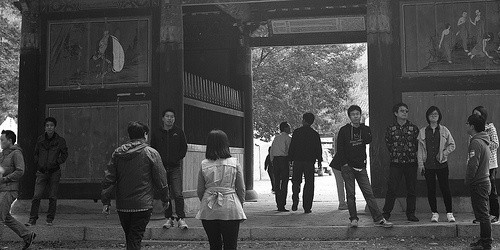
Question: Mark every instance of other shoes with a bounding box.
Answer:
[491,217,499,223]
[382,212,391,220]
[338,204,348,210]
[278,205,290,211]
[292,205,297,211]
[304,209,311,213]
[472,219,480,224]
[408,215,419,222]
[470,242,491,250]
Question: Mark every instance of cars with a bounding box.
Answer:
[315,149,333,175]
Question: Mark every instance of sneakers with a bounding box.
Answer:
[446,213,456,222]
[430,212,440,222]
[373,218,393,228]
[350,219,358,227]
[25,218,36,226]
[178,219,188,230]
[163,217,175,229]
[46,219,53,226]
[22,231,37,250]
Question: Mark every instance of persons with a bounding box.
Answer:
[0,130,37,250]
[288,113,322,213]
[151,109,188,229]
[100,120,171,250]
[196,130,247,250]
[383,103,420,222]
[328,106,393,227]
[265,147,276,193]
[25,117,67,227]
[416,106,455,223]
[465,106,500,250]
[270,121,292,212]
